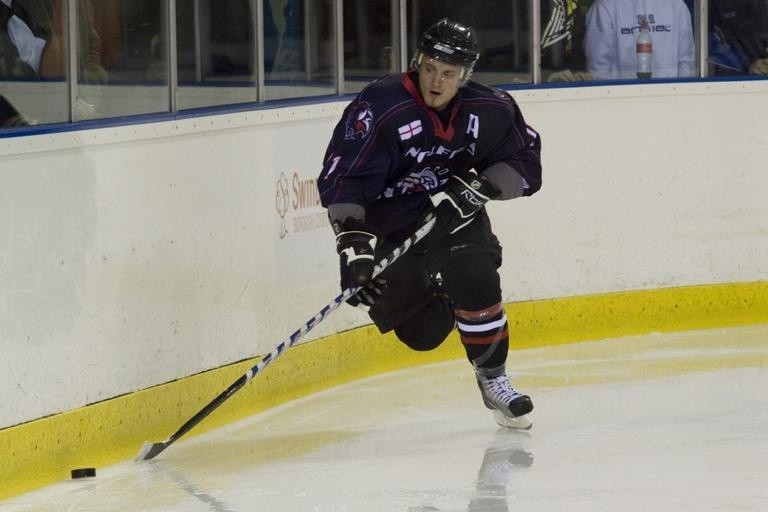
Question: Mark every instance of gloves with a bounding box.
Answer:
[337,230,387,307]
[416,169,500,244]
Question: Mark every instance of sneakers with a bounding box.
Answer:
[474,372,532,418]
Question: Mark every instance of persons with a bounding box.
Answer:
[319,18,552,417]
[540,0,768,88]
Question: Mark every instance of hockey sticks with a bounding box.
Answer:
[135,217,436,462]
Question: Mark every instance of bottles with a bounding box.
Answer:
[636,22,653,79]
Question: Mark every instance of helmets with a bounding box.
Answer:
[410,18,481,89]
